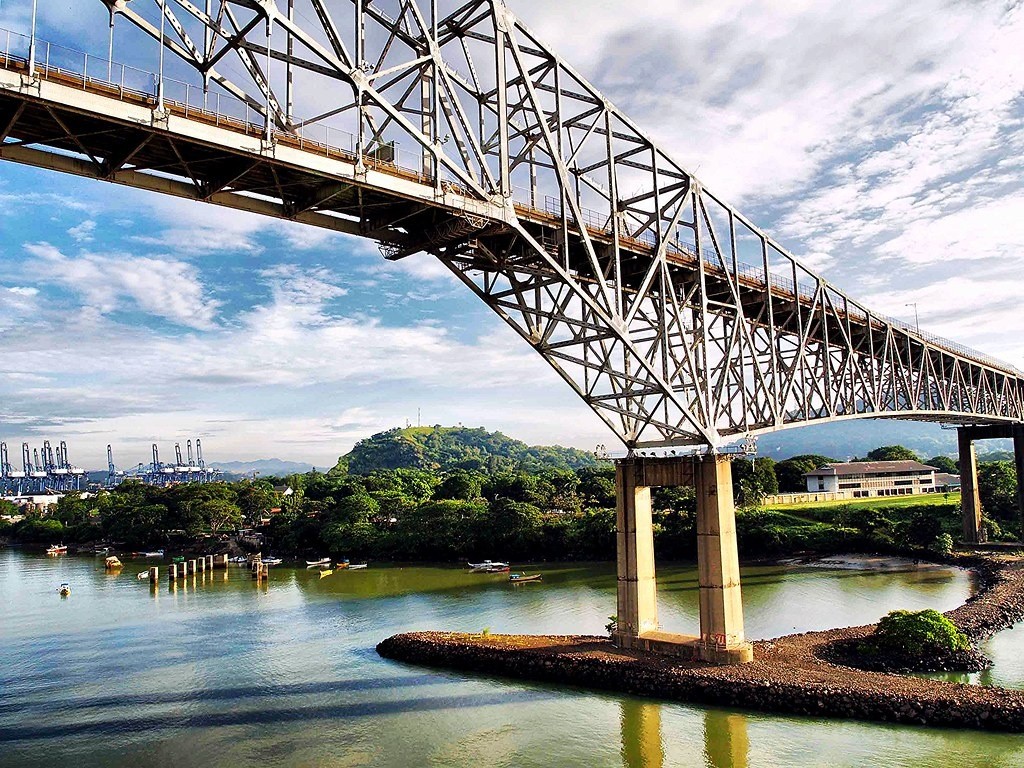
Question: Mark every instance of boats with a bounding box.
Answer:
[468,560,511,572]
[337,563,368,570]
[104,555,124,571]
[229,556,283,567]
[306,556,332,565]
[509,571,543,582]
[59,582,70,597]
[46,544,68,552]
